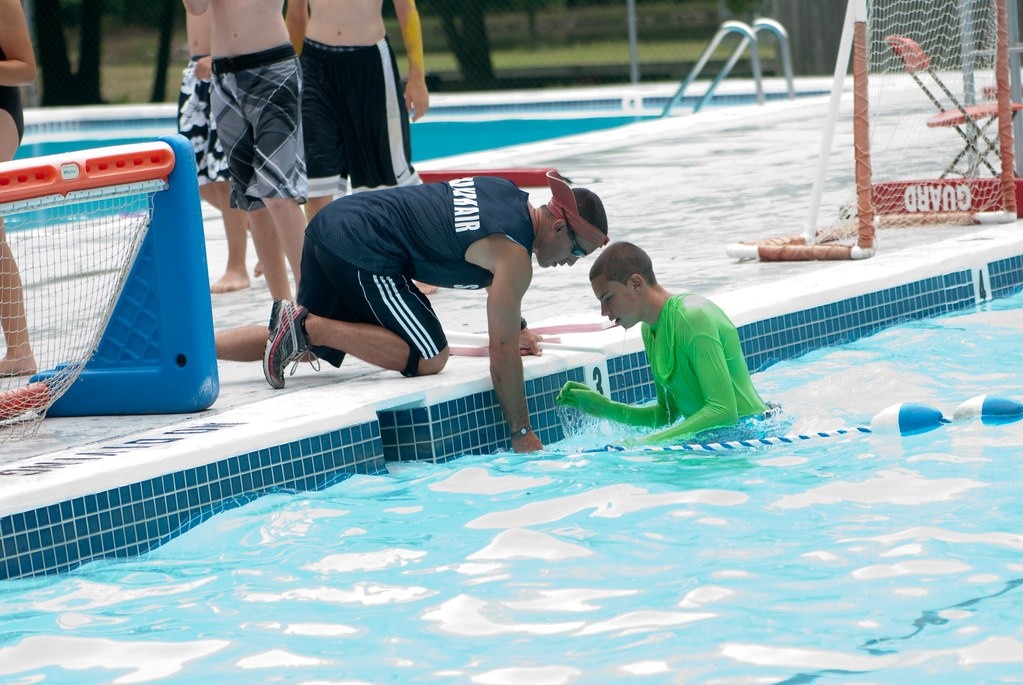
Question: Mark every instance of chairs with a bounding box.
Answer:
[886,33,1022,180]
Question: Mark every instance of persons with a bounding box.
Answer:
[177,0,264,293]
[298,0,429,223]
[217,169,611,453]
[183,0,310,302]
[556,242,770,446]
[0,0,37,377]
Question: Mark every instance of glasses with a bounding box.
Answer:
[561,207,587,257]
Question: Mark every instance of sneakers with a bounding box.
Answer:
[261,298,320,389]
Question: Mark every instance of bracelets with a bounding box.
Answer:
[510,425,532,438]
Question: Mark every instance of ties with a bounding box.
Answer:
[546,170,610,248]
